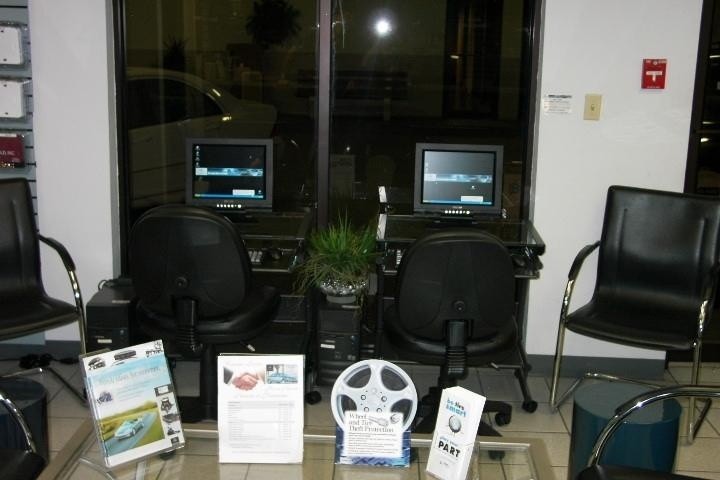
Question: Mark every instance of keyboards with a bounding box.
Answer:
[395,248,404,269]
[248,250,265,263]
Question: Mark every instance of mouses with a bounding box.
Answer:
[269,248,283,260]
[510,253,526,267]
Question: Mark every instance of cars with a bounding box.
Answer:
[266,372,296,384]
[126,66,278,208]
[115,418,144,439]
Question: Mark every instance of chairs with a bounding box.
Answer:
[549,182,720,480]
[377,233,517,459]
[131,207,284,423]
[0,178,92,455]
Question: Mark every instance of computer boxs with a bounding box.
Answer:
[83,283,133,400]
[313,303,361,387]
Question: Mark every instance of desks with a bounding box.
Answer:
[372,209,545,415]
[40,413,557,480]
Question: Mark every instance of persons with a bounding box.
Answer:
[234,369,266,389]
[224,367,258,391]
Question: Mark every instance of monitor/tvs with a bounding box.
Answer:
[413,142,504,229]
[183,137,274,223]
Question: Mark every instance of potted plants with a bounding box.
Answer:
[307,218,371,303]
